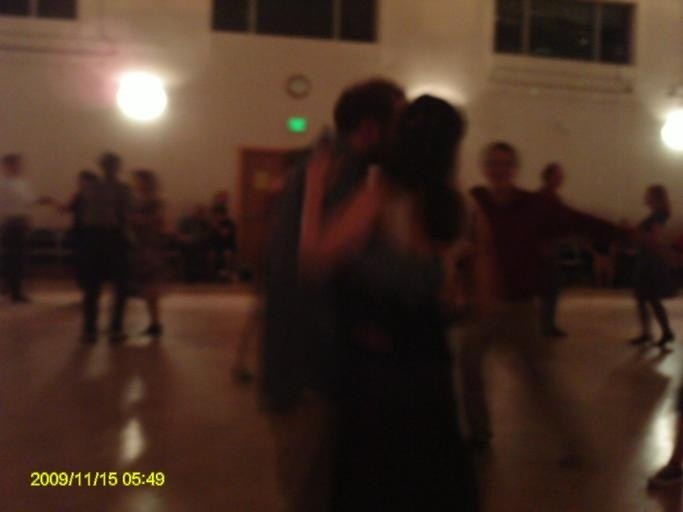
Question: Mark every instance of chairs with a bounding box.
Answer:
[26,225,181,276]
